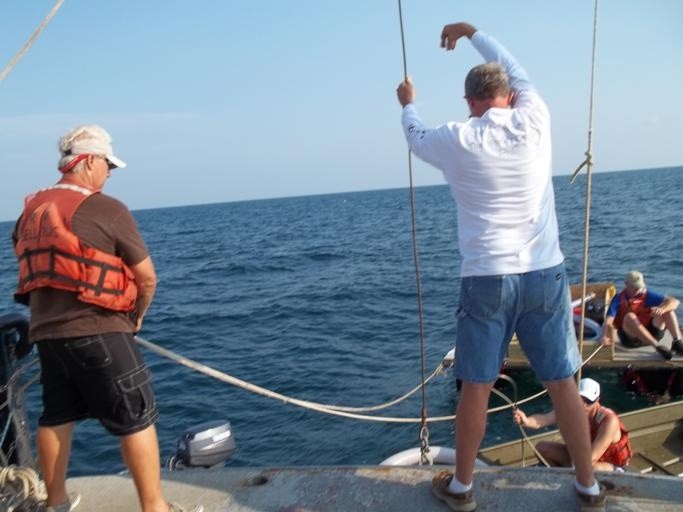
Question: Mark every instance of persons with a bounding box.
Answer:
[512,378,633,473]
[395,22,606,511]
[599,270,682,361]
[11,123,206,511]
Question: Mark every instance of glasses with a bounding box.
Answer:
[582,397,597,405]
[98,156,118,170]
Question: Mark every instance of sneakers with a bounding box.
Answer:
[656,345,673,361]
[432,469,476,512]
[46,492,82,512]
[671,340,683,355]
[575,483,609,512]
[168,502,204,512]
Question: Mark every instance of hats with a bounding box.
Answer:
[576,377,601,401]
[624,270,646,290]
[60,125,127,170]
